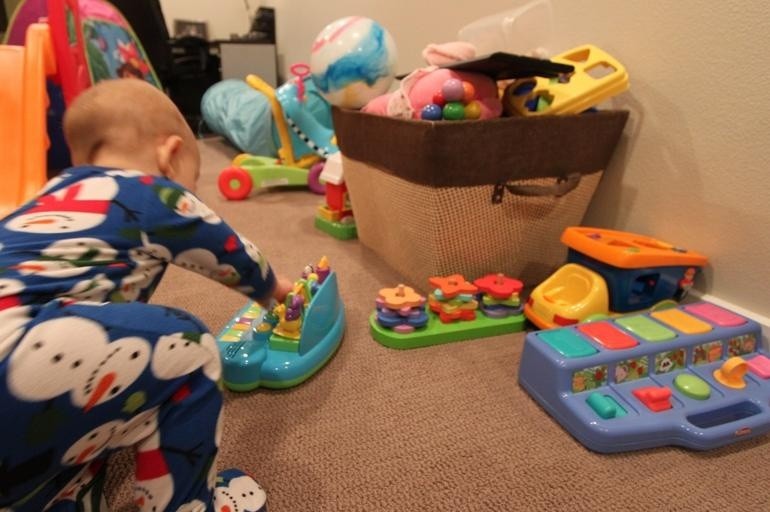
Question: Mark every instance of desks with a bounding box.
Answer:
[168,34,281,122]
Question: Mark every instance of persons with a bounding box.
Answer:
[0,76,293,512]
